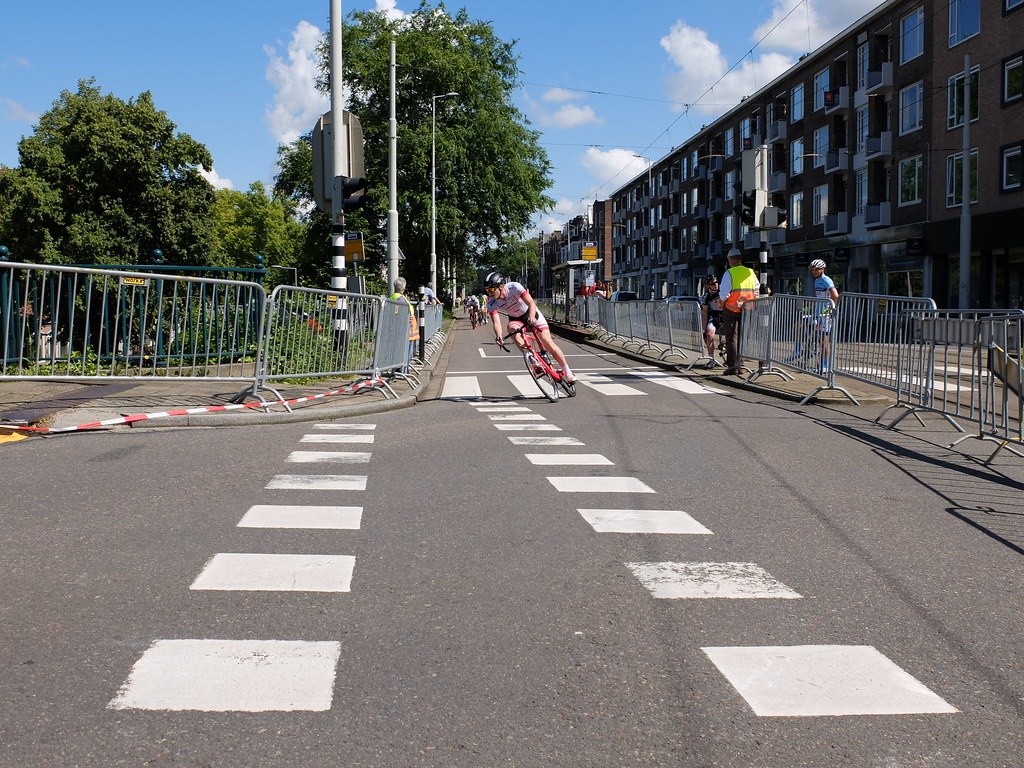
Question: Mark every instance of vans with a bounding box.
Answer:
[609,290,641,301]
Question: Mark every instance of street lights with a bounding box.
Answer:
[428,91,460,303]
[631,154,651,301]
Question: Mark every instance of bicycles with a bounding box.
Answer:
[462,304,489,329]
[498,317,576,401]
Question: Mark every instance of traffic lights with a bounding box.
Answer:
[765,206,787,229]
[742,188,756,225]
[341,176,368,216]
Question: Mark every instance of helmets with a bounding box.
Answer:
[808,259,827,269]
[483,272,504,289]
[703,274,717,283]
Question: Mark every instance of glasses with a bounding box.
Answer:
[485,287,495,292]
[709,281,720,286]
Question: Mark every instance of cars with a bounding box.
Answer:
[664,294,704,312]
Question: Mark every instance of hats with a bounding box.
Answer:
[727,248,741,258]
[471,295,476,300]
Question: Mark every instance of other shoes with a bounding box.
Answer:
[702,361,717,370]
[723,370,738,376]
[812,367,821,375]
[563,371,575,384]
[820,367,830,374]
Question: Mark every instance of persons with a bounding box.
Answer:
[699,273,725,369]
[424,282,444,306]
[719,248,761,376]
[782,258,839,374]
[388,277,420,376]
[462,293,490,329]
[486,271,577,383]
